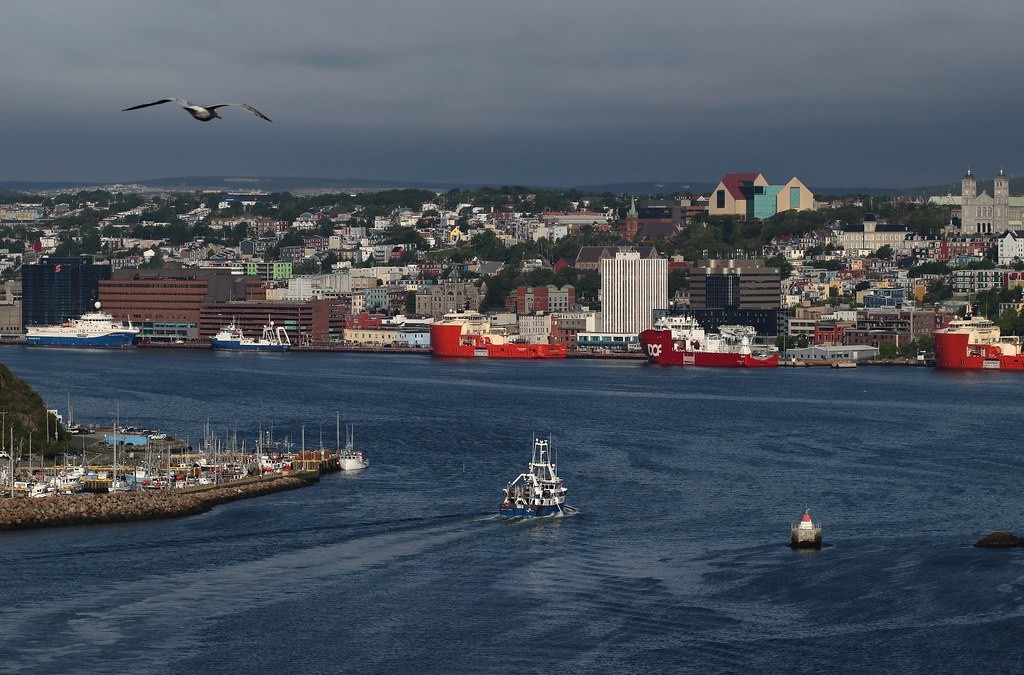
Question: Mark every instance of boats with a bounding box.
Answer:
[791,504,823,550]
[637,303,779,368]
[0,389,290,499]
[208,314,292,352]
[930,303,1024,372]
[337,423,369,471]
[429,300,568,359]
[499,430,568,520]
[24,302,141,350]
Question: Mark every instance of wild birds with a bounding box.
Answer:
[124,98,274,126]
